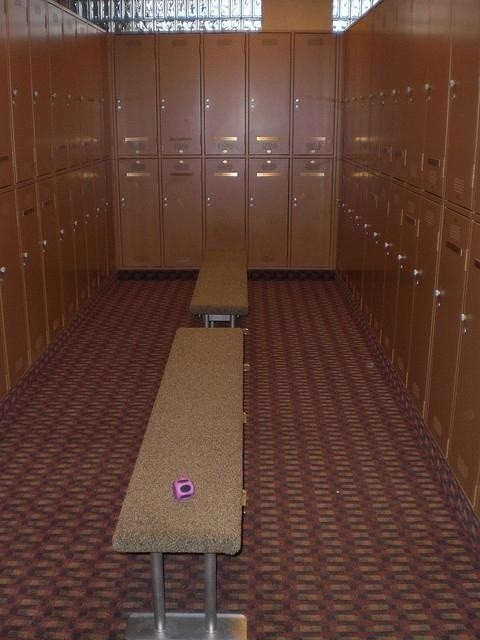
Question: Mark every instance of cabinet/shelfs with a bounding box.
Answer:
[293,33,339,157]
[110,34,159,158]
[155,34,203,155]
[248,159,292,269]
[246,34,292,157]
[204,158,248,268]
[116,156,159,269]
[1,0,109,399]
[341,0,479,520]
[202,34,247,157]
[289,158,336,269]
[161,156,203,268]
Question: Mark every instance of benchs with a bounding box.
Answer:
[111,328,249,640]
[189,271,249,328]
